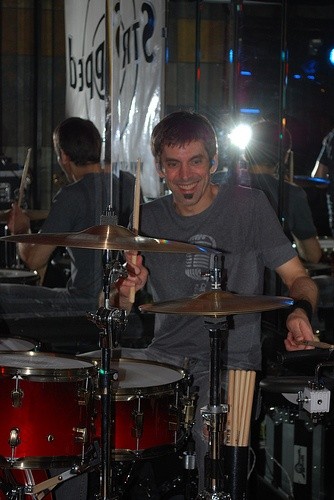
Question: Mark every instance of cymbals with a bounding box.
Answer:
[141,294,293,316]
[0,209,50,223]
[293,176,329,188]
[0,223,222,255]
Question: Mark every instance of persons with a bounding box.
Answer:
[210,120,321,263]
[99,111,319,500]
[12,117,144,353]
[312,129,334,240]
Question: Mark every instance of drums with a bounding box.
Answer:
[304,263,332,276]
[293,238,334,266]
[77,356,194,462]
[0,352,94,469]
[0,337,35,352]
[311,275,334,339]
[256,374,334,500]
[0,267,40,286]
